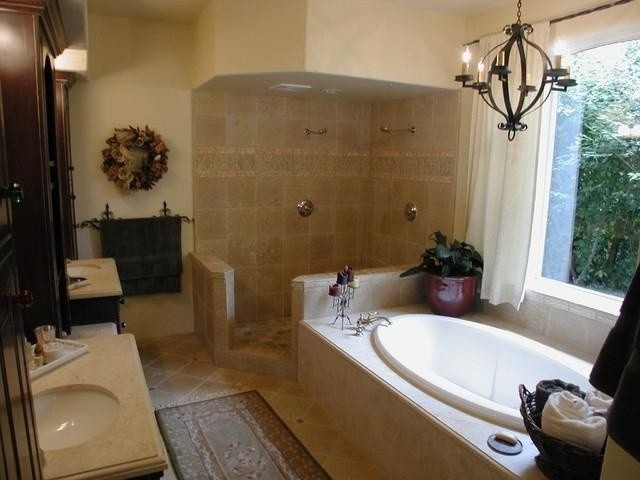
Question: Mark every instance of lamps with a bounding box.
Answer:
[451,0,579,143]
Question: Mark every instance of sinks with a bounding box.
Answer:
[32,382,119,450]
[67,262,101,288]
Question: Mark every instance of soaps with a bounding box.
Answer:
[493,429,517,445]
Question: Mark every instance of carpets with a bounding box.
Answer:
[154,387,333,480]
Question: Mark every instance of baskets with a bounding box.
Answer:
[518,383,605,480]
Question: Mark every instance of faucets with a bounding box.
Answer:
[354,315,392,333]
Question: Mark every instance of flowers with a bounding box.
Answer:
[102,124,169,192]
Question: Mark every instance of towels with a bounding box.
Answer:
[97,216,186,298]
[534,375,610,451]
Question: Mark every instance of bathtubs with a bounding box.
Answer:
[373,312,597,430]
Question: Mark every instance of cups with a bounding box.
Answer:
[32,324,57,352]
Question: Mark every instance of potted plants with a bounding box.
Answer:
[405,228,484,317]
